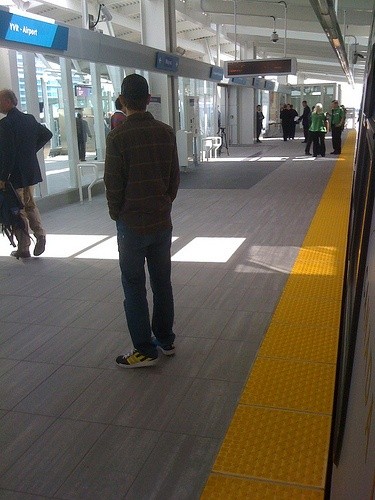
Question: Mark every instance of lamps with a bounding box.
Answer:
[90,3,111,29]
[351,52,359,64]
[13,0,30,10]
[176,47,185,55]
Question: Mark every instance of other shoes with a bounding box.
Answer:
[257,139,260,142]
[302,140,307,143]
[94,156,97,160]
[322,154,325,157]
[330,151,341,154]
[81,159,87,161]
[313,154,317,157]
[305,152,312,155]
[284,138,287,141]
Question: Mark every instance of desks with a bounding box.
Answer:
[177,131,194,168]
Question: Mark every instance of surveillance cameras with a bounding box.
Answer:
[271,33,279,43]
[100,3,112,21]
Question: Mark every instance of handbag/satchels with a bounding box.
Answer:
[0,187,26,229]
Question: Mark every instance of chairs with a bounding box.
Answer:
[200,137,222,161]
[78,160,106,199]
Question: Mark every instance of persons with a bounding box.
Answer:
[103,74,179,368]
[305,103,328,157]
[256,105,264,143]
[104,112,112,137]
[76,113,92,161]
[298,101,312,142]
[280,104,298,140]
[325,100,346,154]
[111,95,127,131]
[0,89,54,257]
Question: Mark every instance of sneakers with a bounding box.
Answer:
[152,336,175,355]
[11,251,31,258]
[34,236,46,256]
[116,348,159,367]
